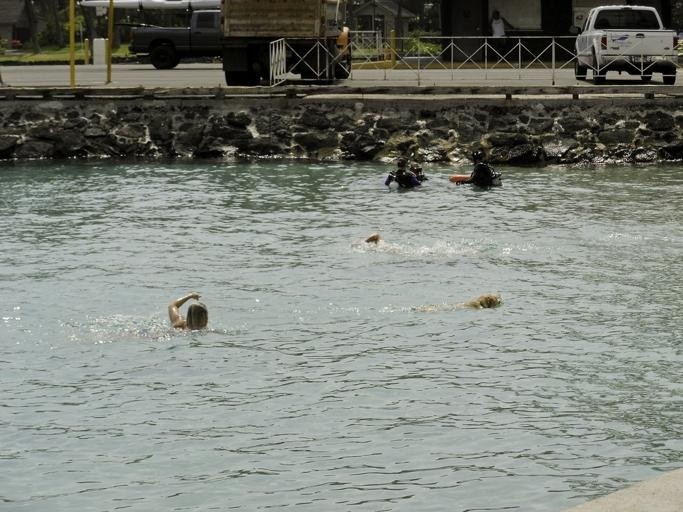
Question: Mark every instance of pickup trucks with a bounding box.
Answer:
[572,7,679,85]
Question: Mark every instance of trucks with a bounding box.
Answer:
[125,6,223,73]
[217,0,357,87]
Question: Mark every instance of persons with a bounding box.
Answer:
[350,234,379,246]
[168,292,208,329]
[410,163,427,181]
[385,158,421,189]
[463,150,492,186]
[490,10,513,63]
